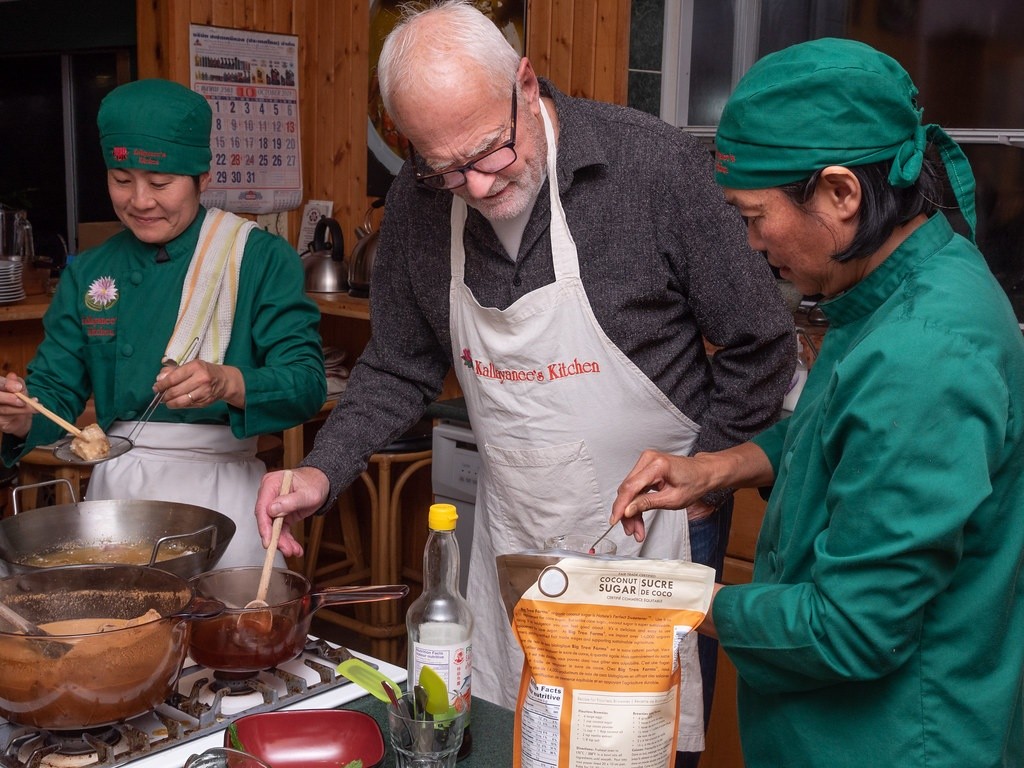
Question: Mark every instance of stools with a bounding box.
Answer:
[13,393,102,508]
[298,423,434,667]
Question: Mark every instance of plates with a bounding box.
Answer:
[0,260,26,302]
[367,0,520,176]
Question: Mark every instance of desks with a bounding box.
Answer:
[0,255,371,389]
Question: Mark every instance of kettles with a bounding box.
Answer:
[348,201,385,298]
[302,219,348,294]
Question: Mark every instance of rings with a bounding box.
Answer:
[188,394,193,401]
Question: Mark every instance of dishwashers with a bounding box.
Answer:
[430,421,480,597]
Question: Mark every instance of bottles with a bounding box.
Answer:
[406,503,472,761]
[45,269,60,295]
[0,209,34,263]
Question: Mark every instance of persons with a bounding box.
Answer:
[255,0,801,768]
[610,36,1024,767]
[0,76,329,607]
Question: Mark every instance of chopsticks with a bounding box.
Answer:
[14,392,88,443]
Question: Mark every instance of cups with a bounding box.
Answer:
[544,534,619,555]
[387,689,468,767]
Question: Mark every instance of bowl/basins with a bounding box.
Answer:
[224,709,384,768]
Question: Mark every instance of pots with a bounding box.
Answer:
[0,479,235,581]
[186,566,408,674]
[0,562,224,730]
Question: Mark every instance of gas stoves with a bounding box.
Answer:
[0,632,408,768]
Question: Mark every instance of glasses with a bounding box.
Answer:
[408,83,518,192]
